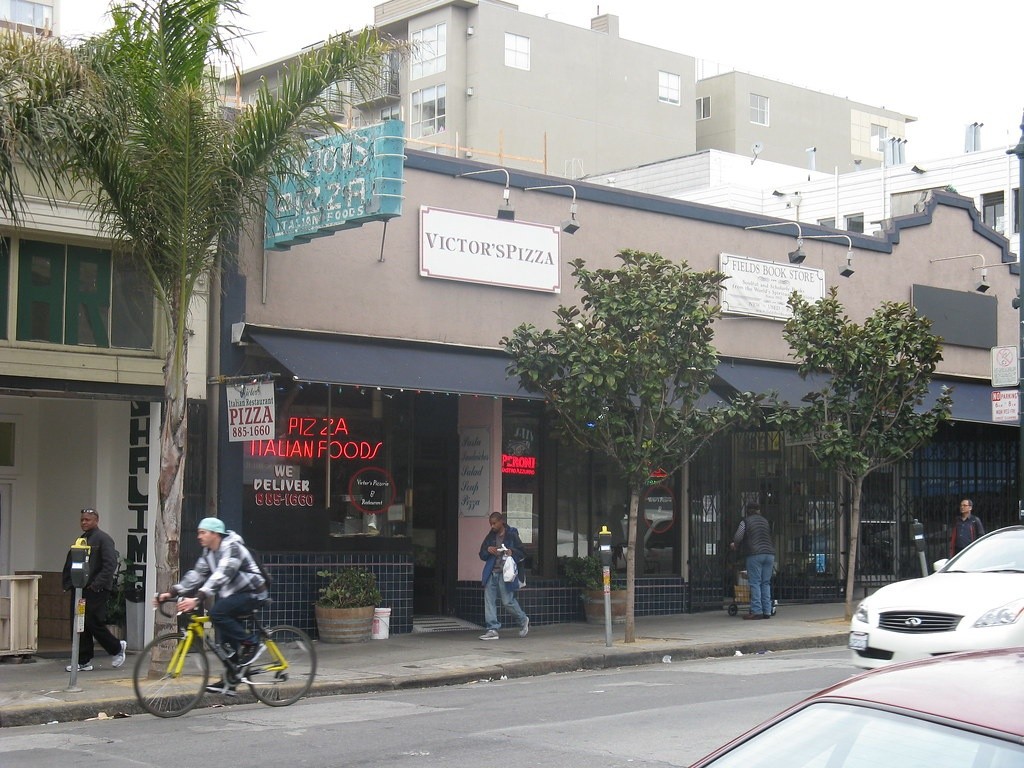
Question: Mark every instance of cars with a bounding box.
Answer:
[507,510,588,552]
[616,509,701,569]
[687,647,1024,768]
[850,525,1024,670]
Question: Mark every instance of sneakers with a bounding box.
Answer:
[519,616,530,638]
[206,680,238,696]
[238,642,268,668]
[478,629,499,639]
[112,639,128,668]
[66,660,93,671]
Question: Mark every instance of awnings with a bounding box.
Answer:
[246,330,737,433]
[712,361,1021,427]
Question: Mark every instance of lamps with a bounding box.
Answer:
[454,168,515,220]
[971,262,1020,298]
[524,184,580,235]
[797,234,856,278]
[745,221,806,264]
[930,254,991,294]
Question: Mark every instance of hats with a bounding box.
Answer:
[198,517,229,535]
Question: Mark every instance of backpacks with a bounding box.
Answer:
[232,541,271,585]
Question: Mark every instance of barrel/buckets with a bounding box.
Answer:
[371,607,392,639]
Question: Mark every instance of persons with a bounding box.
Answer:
[62,510,127,671]
[153,517,270,695]
[952,499,985,555]
[597,504,625,568]
[478,512,529,640]
[730,501,776,620]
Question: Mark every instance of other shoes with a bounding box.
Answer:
[742,611,771,620]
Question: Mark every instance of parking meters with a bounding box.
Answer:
[598,526,613,646]
[65,537,92,692]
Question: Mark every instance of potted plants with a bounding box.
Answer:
[311,568,382,644]
[562,555,627,625]
[93,549,138,643]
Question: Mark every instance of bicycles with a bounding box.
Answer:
[133,593,317,719]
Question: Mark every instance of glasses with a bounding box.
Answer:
[960,504,972,507]
[81,509,98,517]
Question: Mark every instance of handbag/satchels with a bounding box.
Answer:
[496,542,518,582]
